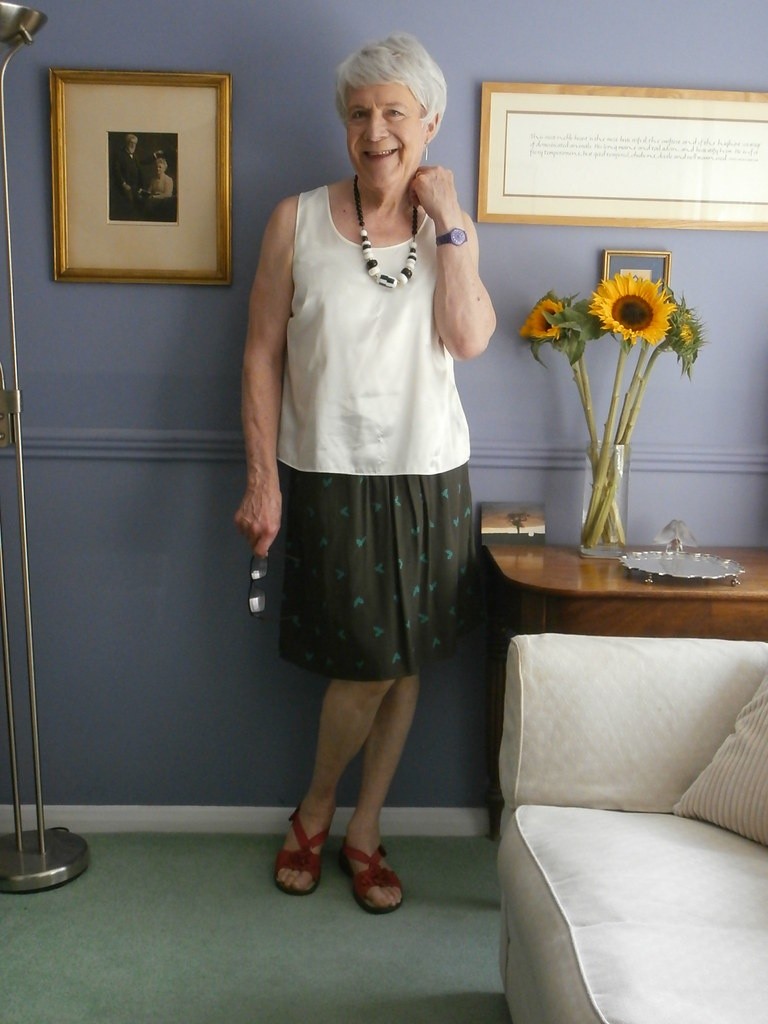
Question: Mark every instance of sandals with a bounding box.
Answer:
[274,805,406,916]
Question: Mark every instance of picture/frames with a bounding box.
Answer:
[602,250,672,304]
[478,84,768,231]
[46,69,231,288]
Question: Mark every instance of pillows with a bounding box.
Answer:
[669,663,768,850]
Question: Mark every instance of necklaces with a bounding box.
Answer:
[353,174,417,289]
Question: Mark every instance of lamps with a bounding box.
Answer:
[0,2,90,894]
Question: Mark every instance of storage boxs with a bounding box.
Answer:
[480,503,545,545]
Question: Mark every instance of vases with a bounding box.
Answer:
[578,445,626,557]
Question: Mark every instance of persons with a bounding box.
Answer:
[232,33,499,915]
[112,133,173,222]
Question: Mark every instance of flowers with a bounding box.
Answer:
[521,279,701,441]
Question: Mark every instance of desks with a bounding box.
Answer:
[489,543,768,637]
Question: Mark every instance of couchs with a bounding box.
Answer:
[496,634,768,1024]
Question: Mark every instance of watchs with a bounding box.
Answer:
[436,228,468,247]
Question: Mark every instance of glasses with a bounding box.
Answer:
[247,550,271,624]
